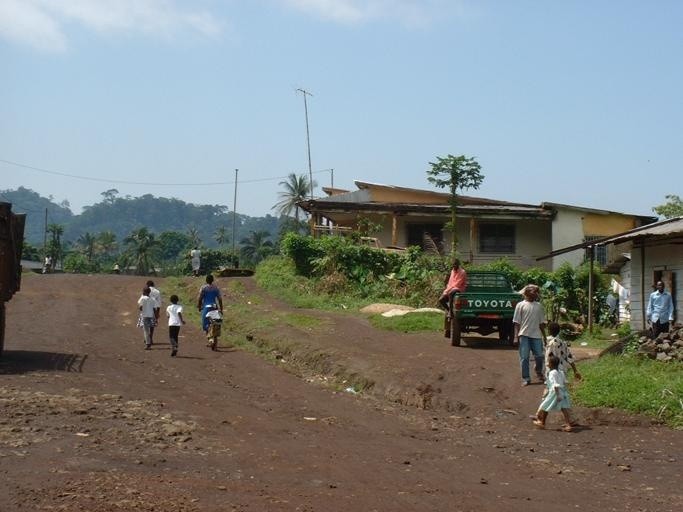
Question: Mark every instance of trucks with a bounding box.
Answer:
[442,269,521,348]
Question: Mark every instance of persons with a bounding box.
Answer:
[438,258,467,319]
[529,322,582,427]
[137,280,160,350]
[196,274,223,336]
[512,285,547,387]
[532,355,572,432]
[191,246,201,276]
[646,280,675,339]
[166,294,186,356]
[45,254,52,273]
[114,263,119,271]
